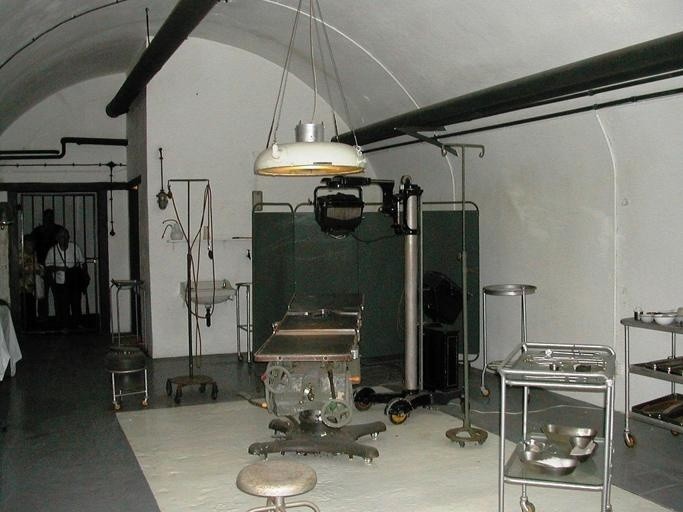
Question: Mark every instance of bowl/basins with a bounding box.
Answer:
[654,314,674,325]
[641,313,654,323]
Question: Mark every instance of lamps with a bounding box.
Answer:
[254,1,368,176]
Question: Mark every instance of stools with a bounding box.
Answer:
[104,355,151,410]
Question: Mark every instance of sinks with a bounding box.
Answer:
[180,278,238,304]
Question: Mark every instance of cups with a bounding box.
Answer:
[634,312,644,321]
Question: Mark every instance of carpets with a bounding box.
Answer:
[116,385,673,511]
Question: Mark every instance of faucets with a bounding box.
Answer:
[223,279,226,288]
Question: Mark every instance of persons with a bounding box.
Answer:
[19,233,44,330]
[33,208,65,317]
[45,228,86,316]
